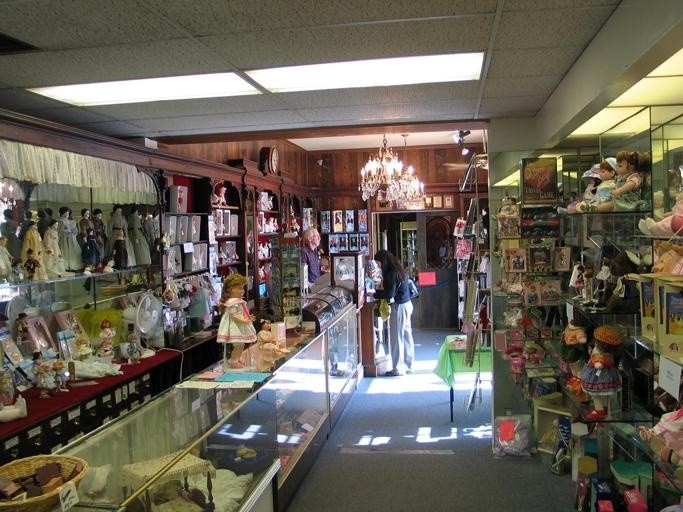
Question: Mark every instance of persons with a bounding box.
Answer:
[328,325,345,376]
[161,183,278,368]
[493,149,683,512]
[367,250,414,376]
[0,182,283,422]
[301,228,331,294]
[1,206,161,406]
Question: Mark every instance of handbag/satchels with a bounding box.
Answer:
[409,278,419,301]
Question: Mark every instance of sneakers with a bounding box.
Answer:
[385,370,396,376]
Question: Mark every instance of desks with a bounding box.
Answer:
[445,332,490,423]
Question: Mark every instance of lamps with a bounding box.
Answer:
[357,134,425,211]
[454,129,472,156]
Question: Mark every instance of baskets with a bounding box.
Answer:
[0,454,89,511]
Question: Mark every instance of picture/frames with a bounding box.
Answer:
[375,192,455,212]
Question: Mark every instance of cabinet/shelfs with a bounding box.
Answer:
[0,106,369,512]
[489,106,683,512]
